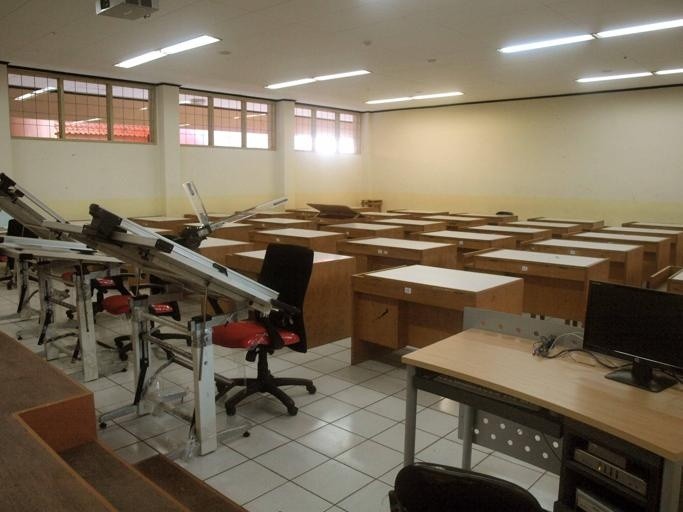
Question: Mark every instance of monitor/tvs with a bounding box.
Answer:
[582,279,683,393]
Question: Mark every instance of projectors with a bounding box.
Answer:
[95,0,160,22]
